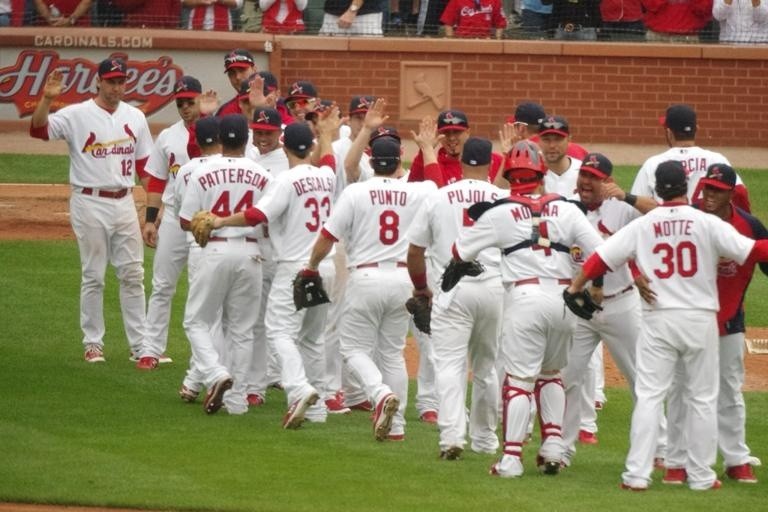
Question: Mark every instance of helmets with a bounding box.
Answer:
[502,141,547,195]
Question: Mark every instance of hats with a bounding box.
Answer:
[98,57,131,80]
[579,153,613,178]
[461,138,492,166]
[658,104,736,194]
[195,71,342,152]
[504,103,546,125]
[437,110,468,133]
[224,48,255,73]
[169,76,202,101]
[537,116,569,137]
[348,95,401,176]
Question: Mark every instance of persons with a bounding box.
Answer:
[629,165,768,484]
[623,103,752,217]
[444,135,604,479]
[0,1,768,48]
[566,160,768,490]
[211,47,258,118]
[140,73,645,455]
[29,57,176,367]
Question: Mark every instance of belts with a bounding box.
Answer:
[603,285,633,299]
[515,278,571,286]
[357,262,407,268]
[208,236,257,242]
[81,187,133,199]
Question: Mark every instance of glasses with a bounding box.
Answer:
[177,101,194,107]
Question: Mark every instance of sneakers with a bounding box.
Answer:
[725,464,759,484]
[620,458,722,491]
[439,434,567,475]
[578,430,599,445]
[84,344,106,362]
[420,411,438,423]
[282,390,406,441]
[595,402,603,410]
[179,376,265,415]
[136,357,158,369]
[128,350,173,363]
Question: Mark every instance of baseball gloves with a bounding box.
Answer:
[295,272,330,310]
[563,289,604,318]
[441,257,484,290]
[191,209,215,245]
[406,295,434,335]
